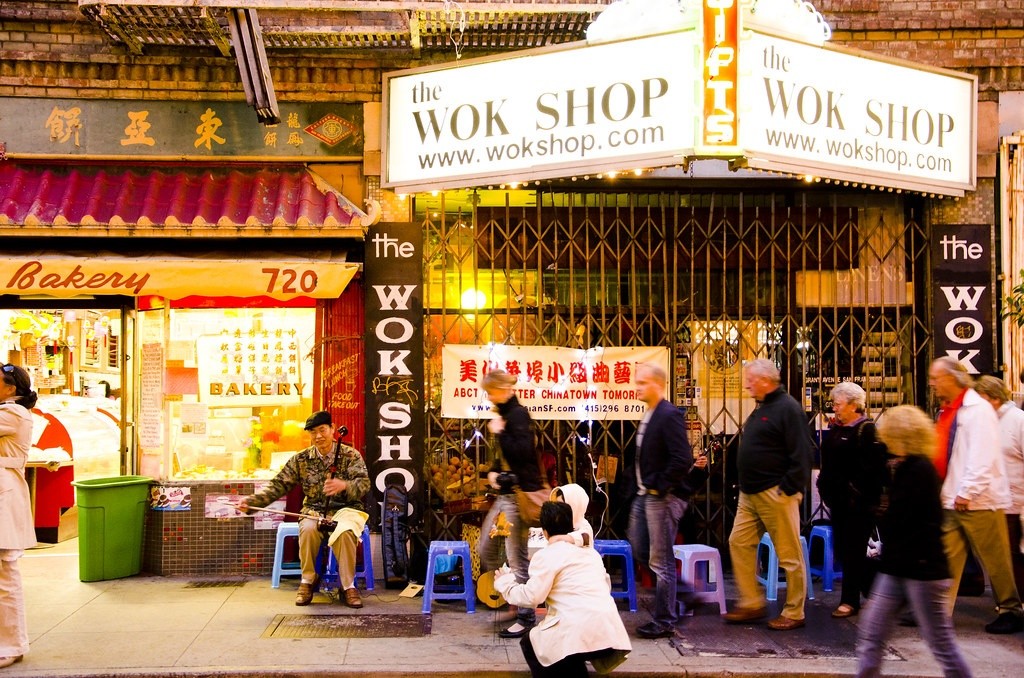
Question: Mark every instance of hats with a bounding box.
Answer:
[303,411,331,431]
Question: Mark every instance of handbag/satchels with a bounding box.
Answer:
[381,484,409,589]
[851,485,893,518]
[517,489,552,527]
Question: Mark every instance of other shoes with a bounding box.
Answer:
[831,603,859,617]
[985,611,1024,633]
[899,612,920,627]
[0,655,23,667]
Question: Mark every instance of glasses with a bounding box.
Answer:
[3,366,17,381]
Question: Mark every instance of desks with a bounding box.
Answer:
[24,461,75,549]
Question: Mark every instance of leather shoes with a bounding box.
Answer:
[767,615,804,629]
[498,623,535,637]
[635,622,674,638]
[722,608,765,621]
[339,586,362,607]
[296,573,319,605]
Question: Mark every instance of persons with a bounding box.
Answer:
[629,364,708,638]
[483,369,548,638]
[548,484,594,547]
[494,501,632,678]
[238,411,370,608]
[816,382,887,617]
[856,404,971,678]
[99,380,114,400]
[0,363,37,667]
[722,360,811,628]
[930,357,1024,634]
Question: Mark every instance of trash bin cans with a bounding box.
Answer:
[70,475,153,583]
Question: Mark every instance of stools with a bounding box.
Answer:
[672,544,727,615]
[422,541,476,614]
[755,532,816,600]
[809,526,843,592]
[593,540,637,611]
[271,522,374,590]
